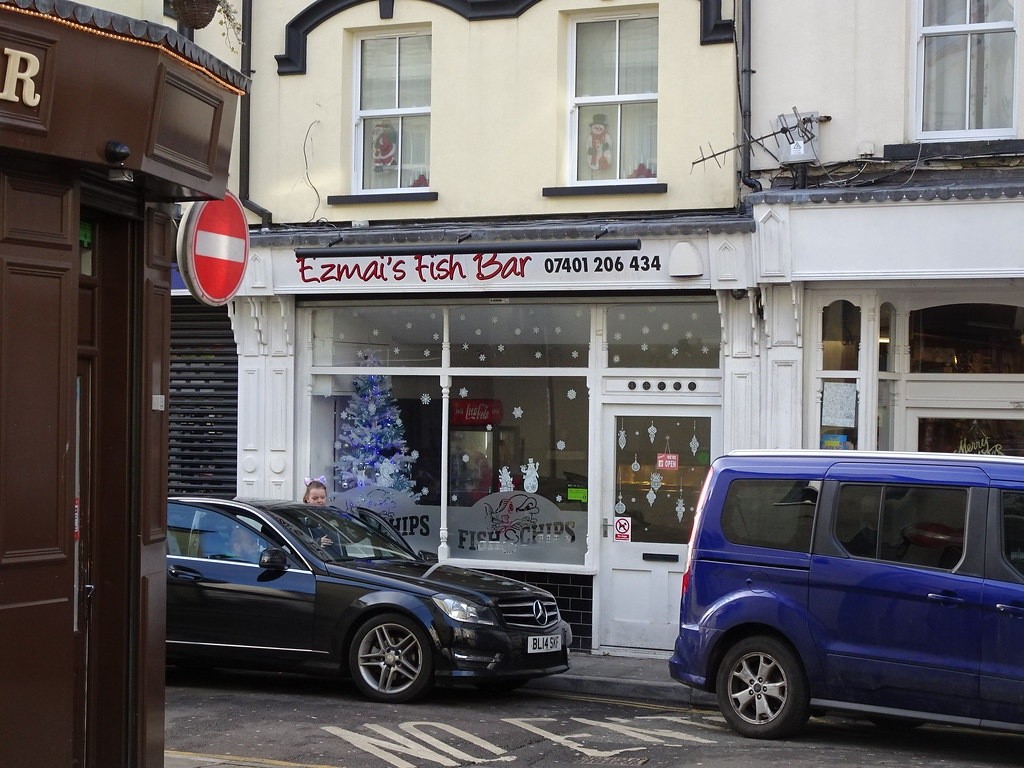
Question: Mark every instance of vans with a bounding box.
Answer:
[667,450,1024,742]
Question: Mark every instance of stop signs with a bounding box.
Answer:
[176,187,250,307]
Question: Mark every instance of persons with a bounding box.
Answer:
[472,441,522,503]
[303,480,370,559]
[225,528,262,565]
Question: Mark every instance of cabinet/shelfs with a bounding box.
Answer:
[450,426,520,506]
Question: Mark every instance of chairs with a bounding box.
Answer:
[168,526,234,559]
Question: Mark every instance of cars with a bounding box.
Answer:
[164,491,574,703]
